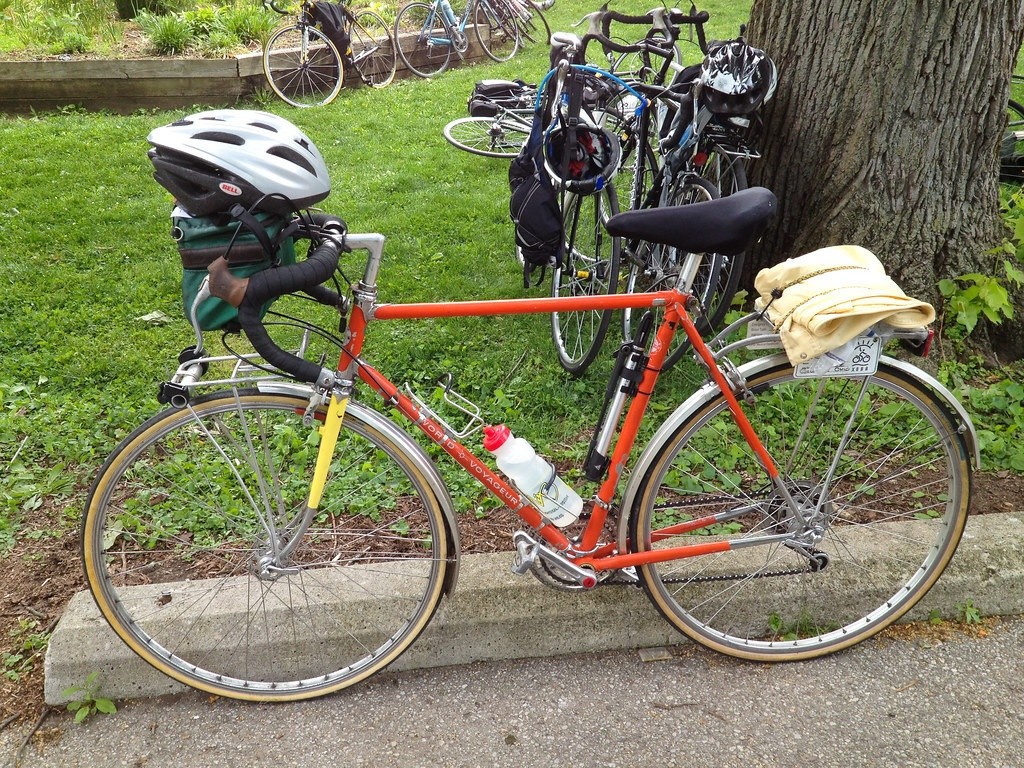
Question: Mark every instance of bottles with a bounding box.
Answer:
[483,424,582,528]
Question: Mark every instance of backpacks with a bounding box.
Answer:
[168,200,297,332]
[507,63,584,289]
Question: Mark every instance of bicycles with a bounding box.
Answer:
[395,1,764,379]
[263,0,397,108]
[1001,74,1024,186]
[80,185,982,704]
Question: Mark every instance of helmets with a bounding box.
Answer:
[541,124,623,196]
[146,108,332,226]
[698,40,779,116]
